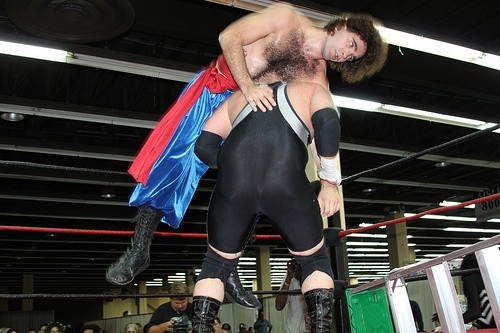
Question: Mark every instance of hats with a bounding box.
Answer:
[222,323,231,330]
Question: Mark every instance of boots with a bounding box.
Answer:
[303,288,335,333]
[225,264,261,309]
[191,295,222,333]
[106,204,164,286]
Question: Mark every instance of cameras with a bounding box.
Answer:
[172,315,191,333]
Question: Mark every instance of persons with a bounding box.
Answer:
[144,281,215,333]
[0,316,272,333]
[389,253,497,333]
[192,80,341,333]
[107,5,387,311]
